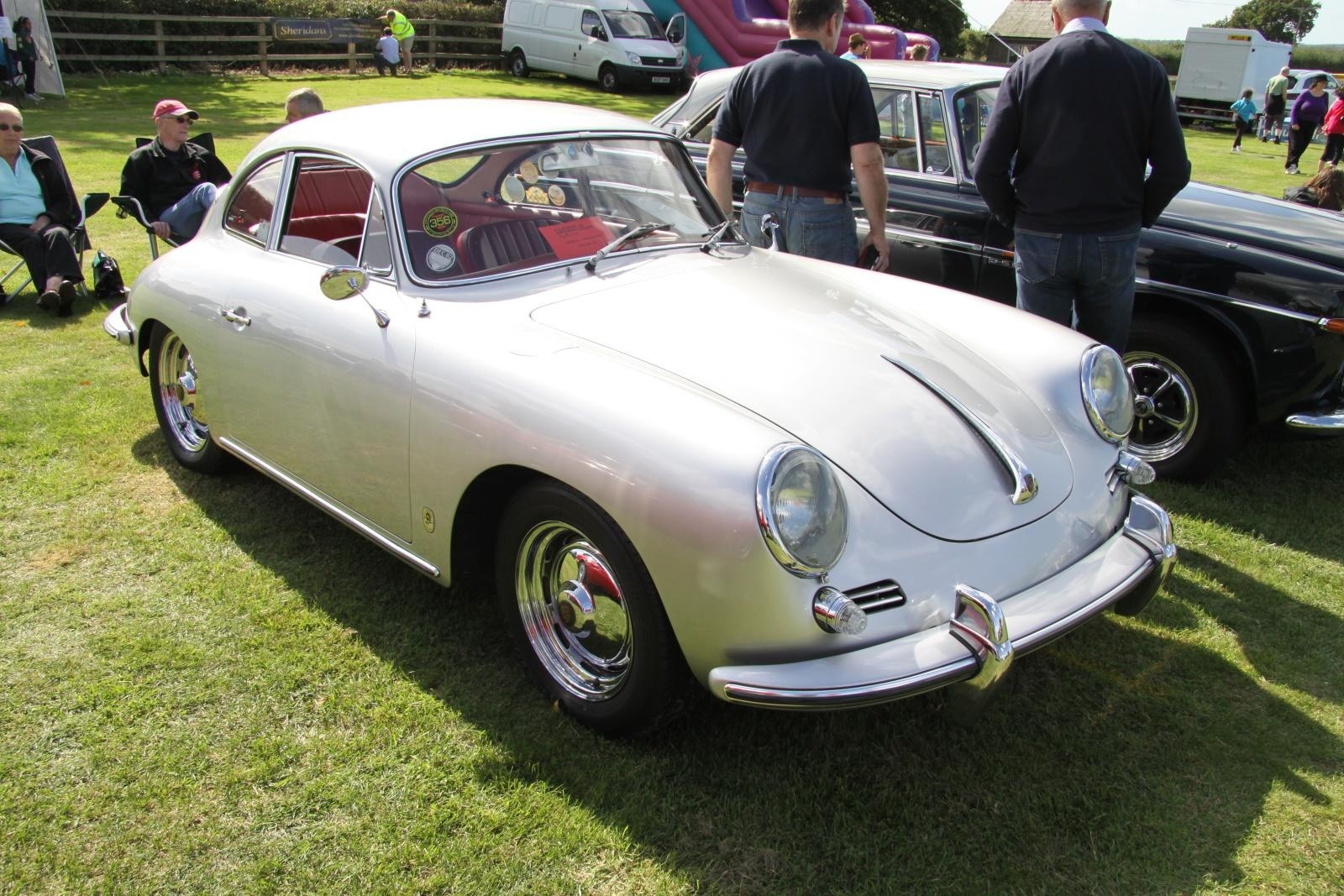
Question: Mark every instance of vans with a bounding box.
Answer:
[502,0,688,93]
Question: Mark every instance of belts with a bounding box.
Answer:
[747,180,846,199]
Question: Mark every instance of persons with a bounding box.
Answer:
[1230,89,1257,151]
[900,45,933,141]
[1263,67,1290,144]
[1283,166,1344,212]
[374,9,415,76]
[973,0,1191,354]
[1318,87,1344,171]
[13,17,45,101]
[1285,75,1330,175]
[120,100,232,240]
[840,33,871,59]
[708,0,890,273]
[286,89,330,123]
[1,102,85,317]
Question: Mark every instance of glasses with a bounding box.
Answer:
[0,123,24,133]
[160,116,194,126]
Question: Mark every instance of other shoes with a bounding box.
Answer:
[1274,138,1280,144]
[36,290,61,313]
[1237,145,1242,152]
[1262,137,1267,142]
[1231,149,1240,154]
[56,280,76,317]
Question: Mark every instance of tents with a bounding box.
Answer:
[0,0,65,97]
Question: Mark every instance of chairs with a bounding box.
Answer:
[456,217,563,279]
[111,130,228,260]
[314,229,449,282]
[0,136,110,303]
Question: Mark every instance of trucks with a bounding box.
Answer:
[1173,26,1293,132]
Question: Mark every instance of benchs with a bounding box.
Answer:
[241,167,442,259]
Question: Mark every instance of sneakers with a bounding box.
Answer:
[1285,164,1300,174]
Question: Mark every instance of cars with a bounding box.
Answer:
[104,97,1178,736]
[591,59,1343,477]
[1282,69,1341,117]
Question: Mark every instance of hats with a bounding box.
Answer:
[153,99,200,121]
[848,33,866,44]
[1313,74,1330,83]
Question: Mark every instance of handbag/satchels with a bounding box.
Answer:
[92,248,126,299]
